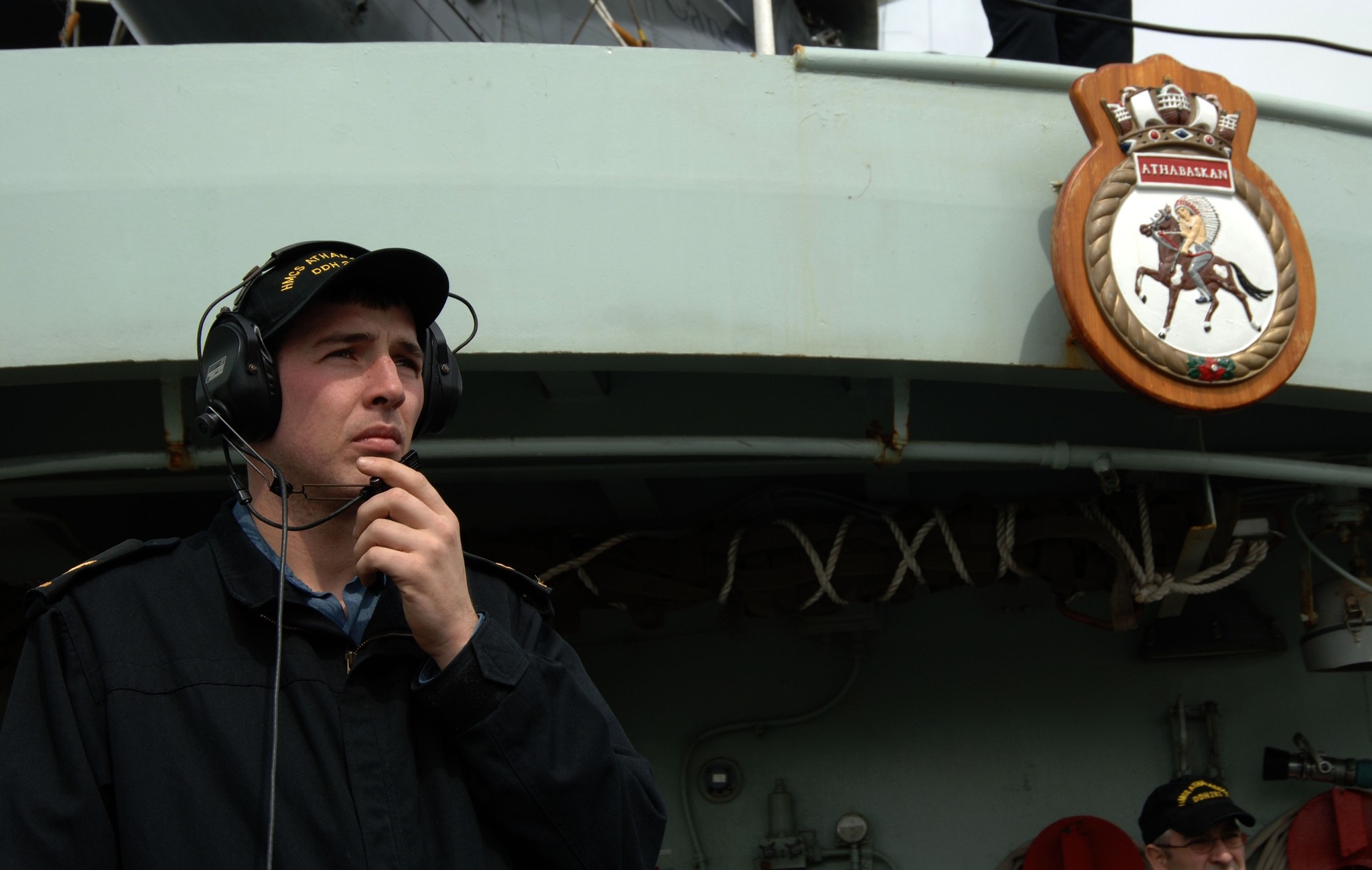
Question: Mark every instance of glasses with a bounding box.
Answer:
[1159,827,1243,855]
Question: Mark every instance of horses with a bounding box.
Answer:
[1135,203,1274,339]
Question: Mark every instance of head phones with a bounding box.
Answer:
[193,240,464,446]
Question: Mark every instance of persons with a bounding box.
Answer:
[0,240,668,870]
[1137,774,1246,870]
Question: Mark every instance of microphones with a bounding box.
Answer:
[220,441,422,532]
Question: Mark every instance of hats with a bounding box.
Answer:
[241,245,450,348]
[1138,775,1255,846]
